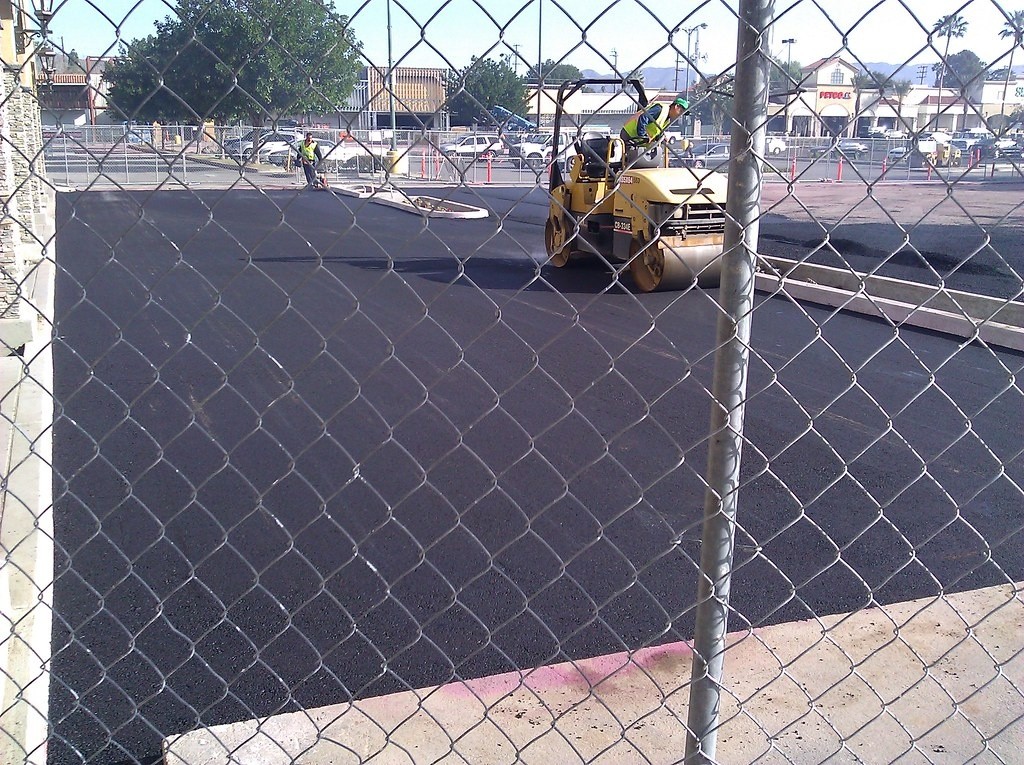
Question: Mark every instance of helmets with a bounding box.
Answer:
[672,98,690,116]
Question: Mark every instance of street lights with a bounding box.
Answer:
[782,37,797,134]
[674,22,708,138]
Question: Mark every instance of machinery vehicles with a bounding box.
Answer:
[545,76,729,295]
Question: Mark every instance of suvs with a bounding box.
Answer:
[432,135,504,163]
[664,125,683,144]
[236,131,308,164]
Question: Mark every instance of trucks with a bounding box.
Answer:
[507,131,615,171]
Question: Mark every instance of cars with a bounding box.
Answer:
[860,125,1024,170]
[810,142,869,160]
[766,136,786,155]
[222,130,252,154]
[668,143,732,168]
[262,138,347,163]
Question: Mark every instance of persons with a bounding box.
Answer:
[620,98,690,145]
[297,132,325,187]
[68,48,80,66]
